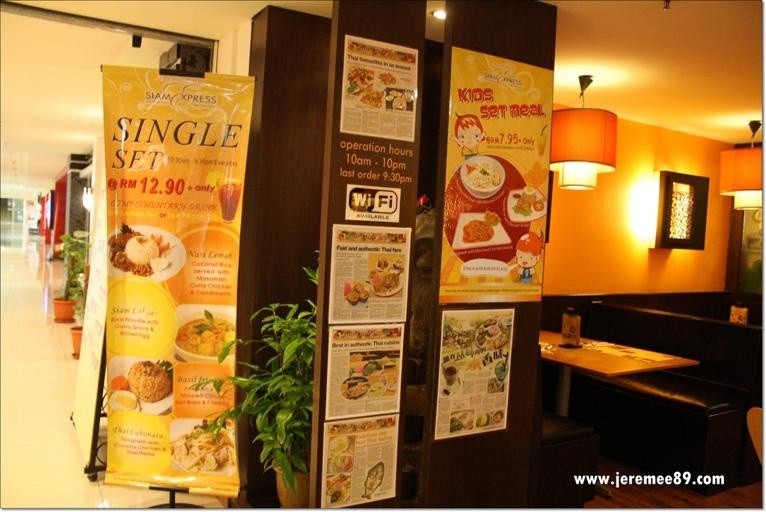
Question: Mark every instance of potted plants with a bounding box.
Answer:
[66,278,87,360]
[48,230,92,324]
[182,249,322,507]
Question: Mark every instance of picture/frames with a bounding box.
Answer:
[547,169,554,244]
[652,171,709,250]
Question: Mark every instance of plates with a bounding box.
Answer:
[168,435,234,473]
[505,186,547,224]
[369,270,404,297]
[340,367,397,400]
[106,224,187,283]
[451,210,512,251]
[324,435,352,506]
[106,364,173,416]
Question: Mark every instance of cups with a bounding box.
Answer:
[441,362,460,386]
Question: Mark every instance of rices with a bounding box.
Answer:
[124,235,158,265]
[127,360,171,402]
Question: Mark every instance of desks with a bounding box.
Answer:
[538,326,702,499]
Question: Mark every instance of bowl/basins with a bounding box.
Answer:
[459,155,506,199]
[173,317,234,365]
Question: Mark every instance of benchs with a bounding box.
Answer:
[574,293,761,496]
[534,408,608,504]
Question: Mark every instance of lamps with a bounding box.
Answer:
[548,73,619,193]
[716,120,762,212]
